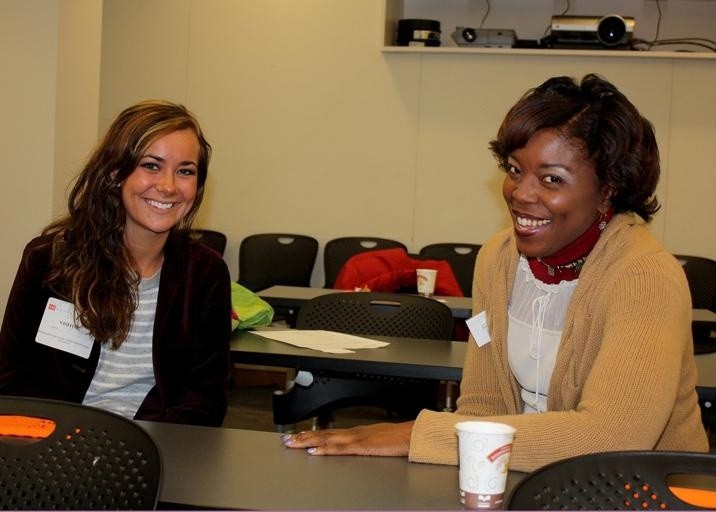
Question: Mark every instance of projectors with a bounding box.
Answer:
[451,27,517,49]
[541,14,636,50]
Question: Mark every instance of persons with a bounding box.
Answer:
[279,72,710,474]
[0,98,233,429]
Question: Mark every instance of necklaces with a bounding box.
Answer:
[534,253,588,278]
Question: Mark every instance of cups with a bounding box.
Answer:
[453,420,517,512]
[414,269,438,298]
